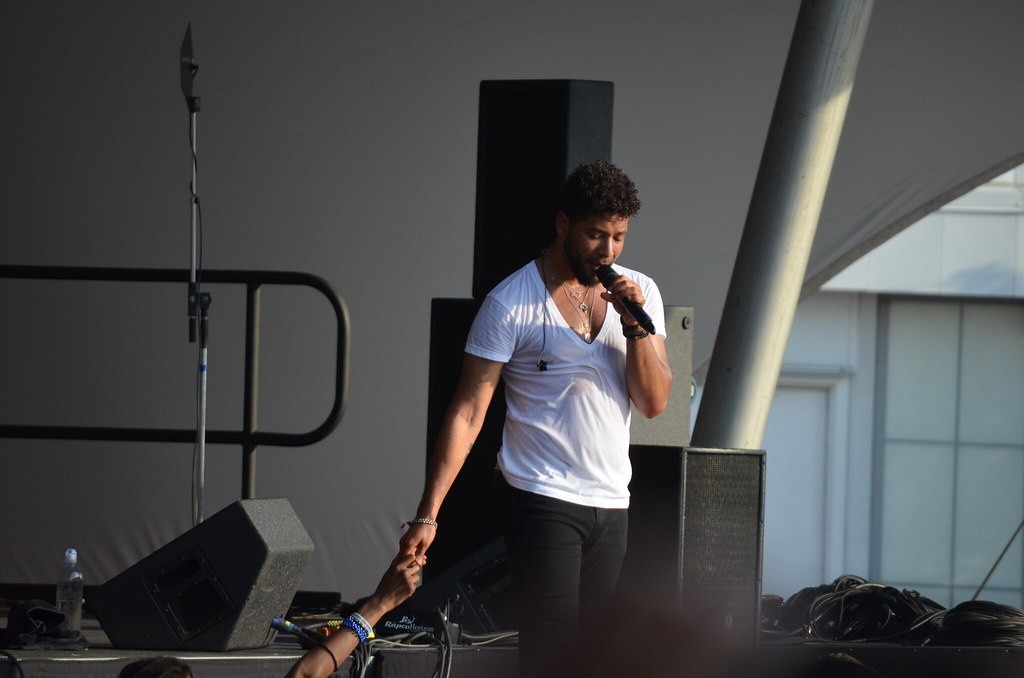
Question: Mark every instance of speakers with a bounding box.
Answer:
[472,80,614,308]
[85,497,314,651]
[427,296,510,577]
[626,301,697,445]
[606,442,767,660]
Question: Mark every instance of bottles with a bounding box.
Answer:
[56,549,83,631]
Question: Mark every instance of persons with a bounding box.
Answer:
[401,163,671,678]
[118,552,421,678]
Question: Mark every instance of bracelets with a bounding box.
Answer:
[401,519,438,528]
[620,316,648,340]
[339,614,375,644]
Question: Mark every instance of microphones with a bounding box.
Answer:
[596,264,656,335]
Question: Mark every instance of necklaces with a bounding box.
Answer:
[546,257,597,343]
